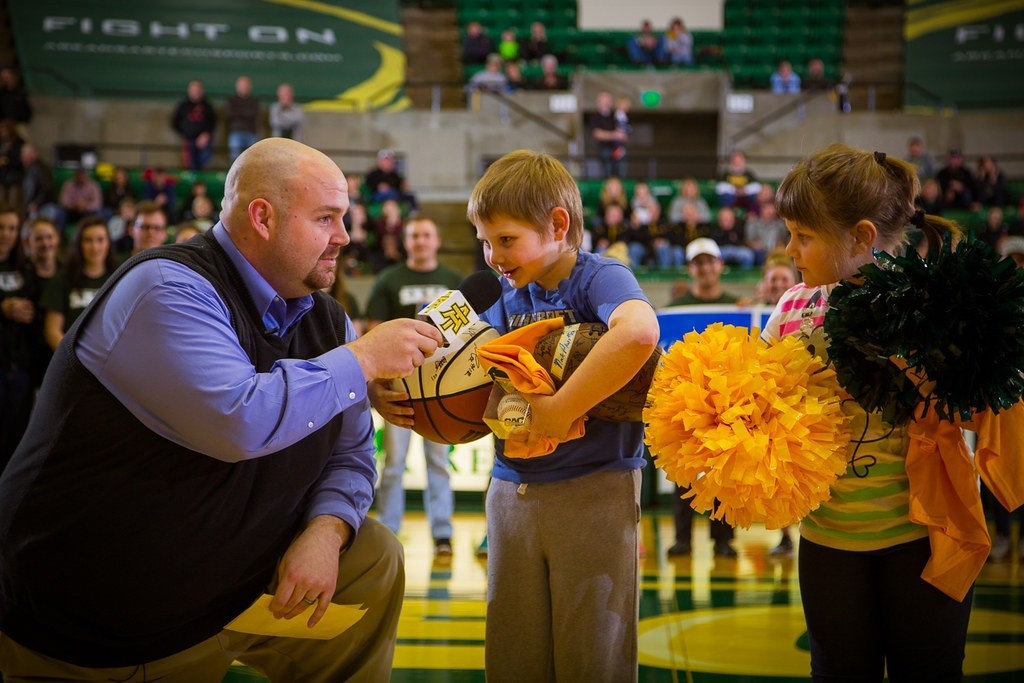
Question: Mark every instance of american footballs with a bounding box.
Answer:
[533,321,669,423]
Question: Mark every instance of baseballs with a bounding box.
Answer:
[497,393,531,433]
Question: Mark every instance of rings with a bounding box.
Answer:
[301,598,314,605]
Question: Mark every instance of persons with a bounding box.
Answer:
[0,65,37,139]
[455,12,1024,274]
[0,140,418,417]
[269,82,304,142]
[657,238,744,561]
[361,213,465,556]
[0,137,444,683]
[365,147,661,683]
[224,73,261,164]
[729,141,989,682]
[168,79,217,171]
[755,257,802,557]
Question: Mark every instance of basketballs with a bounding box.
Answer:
[387,319,502,446]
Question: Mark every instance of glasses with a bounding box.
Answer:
[137,225,163,232]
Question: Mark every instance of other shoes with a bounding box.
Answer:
[713,542,736,555]
[436,539,453,554]
[669,541,691,553]
[769,540,793,554]
[478,539,488,554]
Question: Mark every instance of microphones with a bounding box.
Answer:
[416,270,502,344]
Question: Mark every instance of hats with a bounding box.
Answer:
[685,237,721,261]
